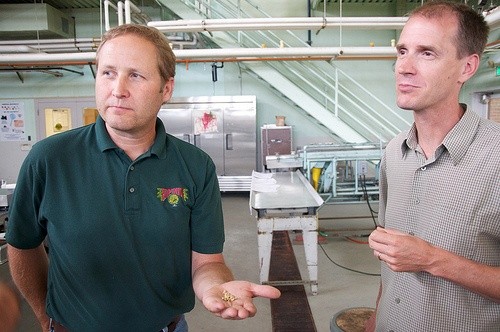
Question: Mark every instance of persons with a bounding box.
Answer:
[0,278,23,332]
[364,0,499,332]
[4,24,281,332]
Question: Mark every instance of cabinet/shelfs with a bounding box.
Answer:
[157,95,224,191]
[303,144,388,204]
[224,95,257,191]
[261,124,292,173]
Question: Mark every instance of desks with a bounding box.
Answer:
[249,169,325,295]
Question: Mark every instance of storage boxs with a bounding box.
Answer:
[275,115,285,127]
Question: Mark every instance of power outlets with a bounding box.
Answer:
[360,163,368,174]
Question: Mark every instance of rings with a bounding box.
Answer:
[377,253,382,261]
